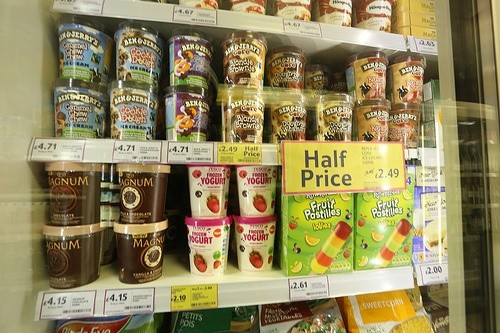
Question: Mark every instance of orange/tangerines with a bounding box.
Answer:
[290,192,411,273]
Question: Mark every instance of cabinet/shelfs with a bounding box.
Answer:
[0,1,468,333]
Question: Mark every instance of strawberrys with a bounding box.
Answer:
[194,195,267,272]
